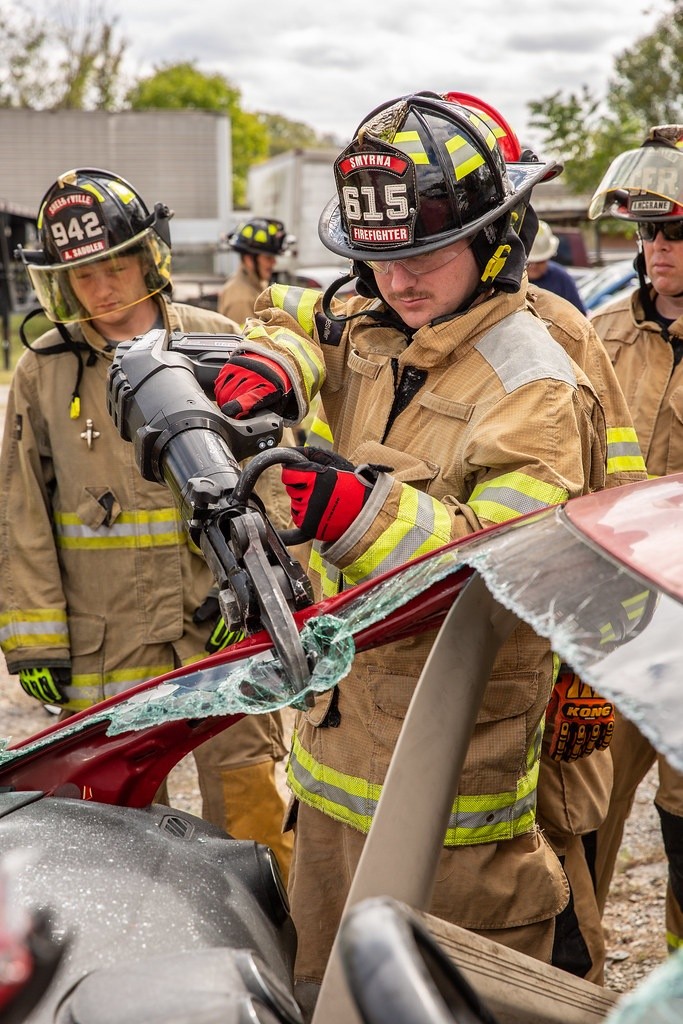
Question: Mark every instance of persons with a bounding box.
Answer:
[212,92,655,1024]
[219,124,683,955]
[0,168,303,891]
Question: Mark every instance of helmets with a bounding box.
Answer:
[318,90,563,261]
[229,219,298,256]
[14,168,174,323]
[588,124,683,219]
[439,91,520,182]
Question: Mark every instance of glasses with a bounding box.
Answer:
[359,233,478,276]
[638,219,683,242]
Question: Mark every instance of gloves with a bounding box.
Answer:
[18,666,72,705]
[280,445,394,542]
[541,662,615,763]
[213,349,293,422]
[204,597,248,656]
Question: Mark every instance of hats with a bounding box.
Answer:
[527,220,560,263]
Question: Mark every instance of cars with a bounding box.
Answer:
[0,472,683,1023]
[549,225,650,317]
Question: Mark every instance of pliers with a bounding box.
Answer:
[104,327,328,711]
